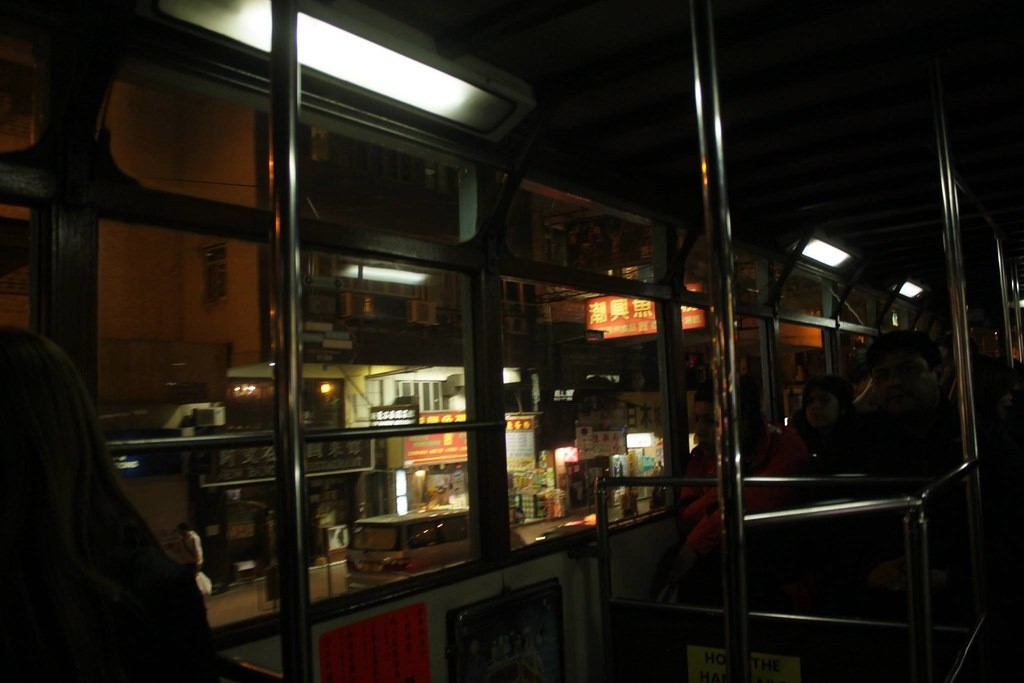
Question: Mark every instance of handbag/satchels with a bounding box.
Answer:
[197,571,212,597]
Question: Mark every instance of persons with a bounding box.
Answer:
[789,327,1024,683]
[0,322,221,683]
[651,376,815,624]
[176,523,203,579]
[619,461,664,518]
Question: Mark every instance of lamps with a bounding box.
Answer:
[799,237,856,275]
[888,277,929,303]
[154,0,538,144]
